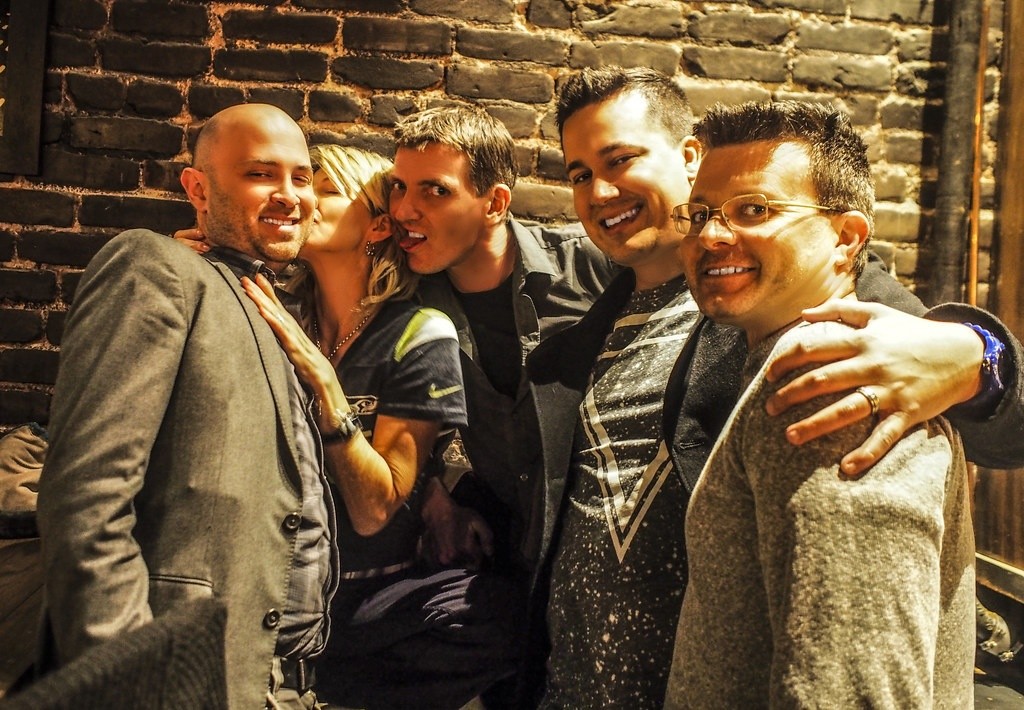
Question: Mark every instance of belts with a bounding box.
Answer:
[269,657,322,692]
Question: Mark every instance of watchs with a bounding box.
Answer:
[955,322,1011,408]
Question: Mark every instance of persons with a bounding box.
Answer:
[36,105,341,710]
[658,100,977,710]
[174,145,469,709]
[515,66,1024,710]
[388,106,622,709]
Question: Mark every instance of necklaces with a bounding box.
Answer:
[314,313,372,359]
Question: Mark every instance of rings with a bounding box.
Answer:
[858,385,879,414]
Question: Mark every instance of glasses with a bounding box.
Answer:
[670,194,846,237]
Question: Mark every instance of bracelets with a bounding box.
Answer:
[323,412,361,447]
